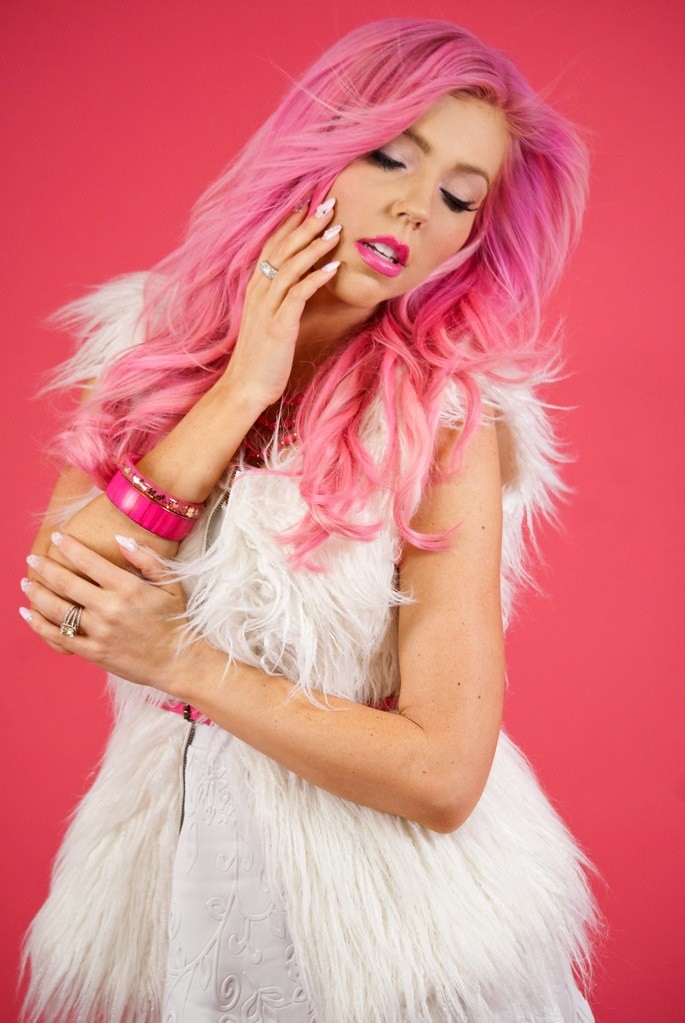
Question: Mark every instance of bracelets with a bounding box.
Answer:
[105,451,205,541]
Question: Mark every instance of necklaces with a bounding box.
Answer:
[239,395,302,459]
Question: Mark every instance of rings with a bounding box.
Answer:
[260,259,278,279]
[61,605,82,636]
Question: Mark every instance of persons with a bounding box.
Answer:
[18,17,601,1022]
